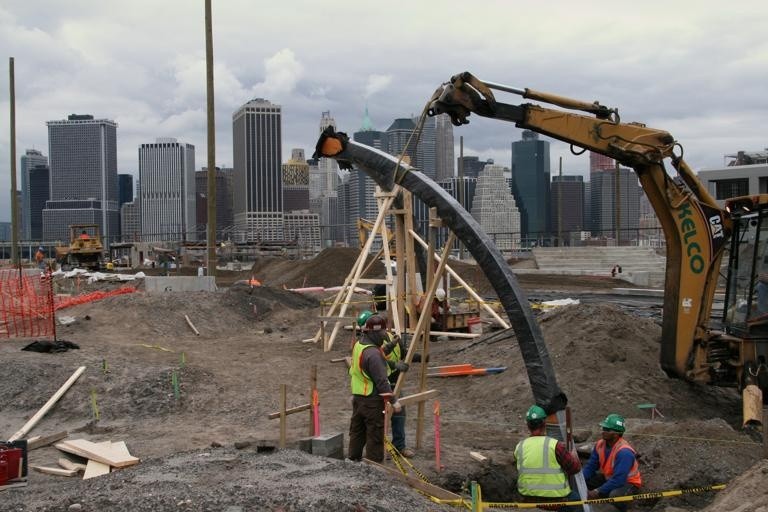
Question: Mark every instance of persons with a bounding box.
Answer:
[416,287,452,342]
[616,264,623,273]
[611,265,617,277]
[581,412,643,511]
[34,244,47,269]
[347,313,402,464]
[78,230,91,239]
[511,404,581,511]
[357,309,416,458]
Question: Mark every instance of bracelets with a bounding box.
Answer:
[594,488,600,496]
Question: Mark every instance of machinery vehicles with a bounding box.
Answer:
[51,223,105,275]
[422,67,768,408]
[355,215,398,265]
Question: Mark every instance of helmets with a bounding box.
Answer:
[598,414,626,432]
[526,405,547,421]
[357,310,379,326]
[366,315,386,330]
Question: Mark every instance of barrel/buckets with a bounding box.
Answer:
[0,439,28,486]
[468,317,482,335]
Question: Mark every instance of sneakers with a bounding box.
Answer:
[395,447,415,458]
